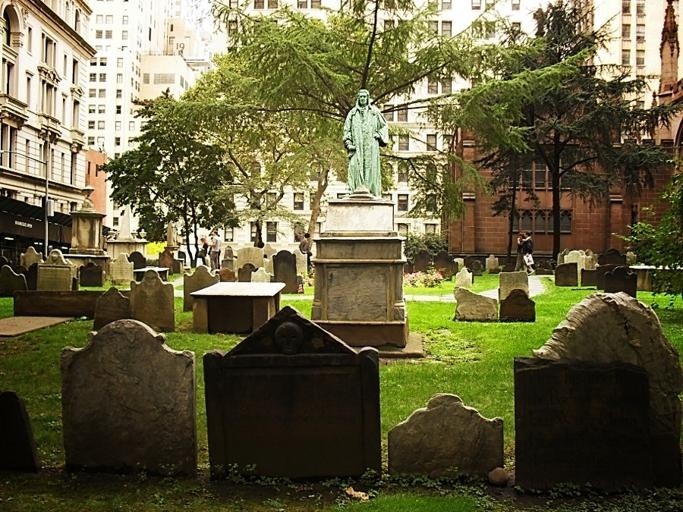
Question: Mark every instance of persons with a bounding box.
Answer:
[198,230,221,271]
[514,233,531,273]
[342,89,389,198]
[299,233,312,271]
[522,232,535,276]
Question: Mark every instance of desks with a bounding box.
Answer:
[190,282,286,333]
[629,265,683,291]
[133,267,169,281]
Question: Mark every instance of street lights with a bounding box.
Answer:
[1,150,50,258]
